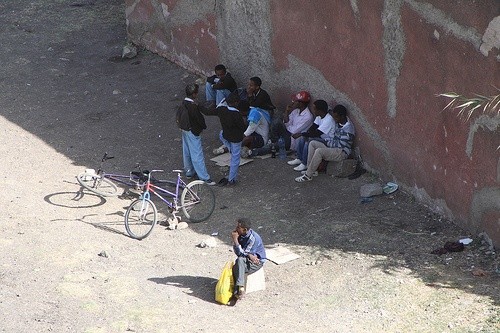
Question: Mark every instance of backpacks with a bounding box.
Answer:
[258,106,285,143]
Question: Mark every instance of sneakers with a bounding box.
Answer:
[287,158,302,165]
[293,162,307,171]
[301,168,319,176]
[294,173,313,182]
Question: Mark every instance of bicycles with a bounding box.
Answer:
[76,151,187,198]
[125,163,216,240]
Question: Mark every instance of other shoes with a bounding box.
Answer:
[187,176,192,179]
[213,148,225,154]
[227,293,239,305]
[204,101,216,109]
[286,149,302,158]
[226,180,238,186]
[205,179,217,186]
[240,147,254,158]
[237,285,244,294]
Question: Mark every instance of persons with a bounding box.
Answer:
[246,78,276,116]
[288,99,336,172]
[219,95,246,185]
[242,90,315,158]
[212,103,270,154]
[203,64,238,116]
[228,217,266,306]
[177,84,217,184]
[294,104,356,182]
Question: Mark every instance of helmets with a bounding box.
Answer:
[291,90,311,103]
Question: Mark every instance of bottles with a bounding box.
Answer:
[278,135,287,158]
[271,143,276,158]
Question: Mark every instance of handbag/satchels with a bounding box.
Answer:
[214,261,235,305]
